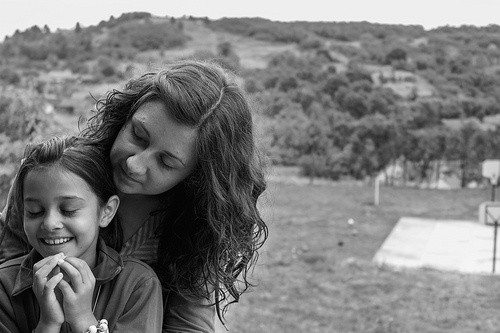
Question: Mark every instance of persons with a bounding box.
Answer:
[0,58,270,333]
[1,133,165,333]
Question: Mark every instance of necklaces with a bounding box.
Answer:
[120,201,166,242]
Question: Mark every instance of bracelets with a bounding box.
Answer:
[85,318,110,333]
